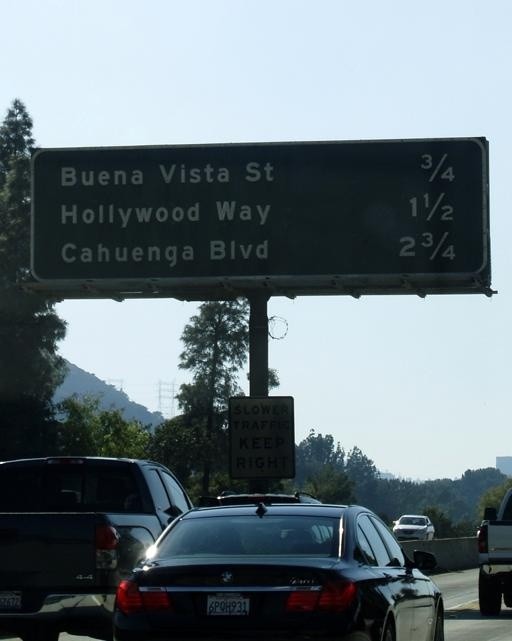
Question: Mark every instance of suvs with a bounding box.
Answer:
[197,486,325,506]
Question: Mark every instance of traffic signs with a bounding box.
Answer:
[228,394,294,479]
[31,137,487,291]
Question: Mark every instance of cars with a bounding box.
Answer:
[116,492,445,641]
[393,511,435,542]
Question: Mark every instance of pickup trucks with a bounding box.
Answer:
[0,454,192,640]
[478,485,512,620]
